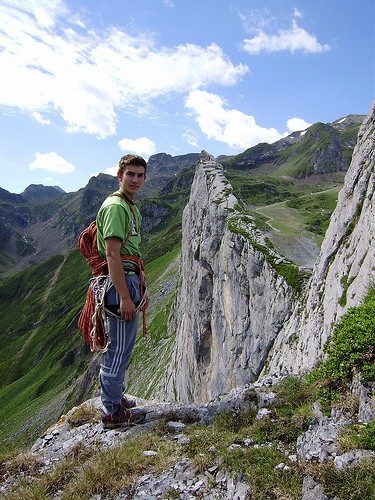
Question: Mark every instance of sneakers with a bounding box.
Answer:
[121,399,138,409]
[103,408,147,428]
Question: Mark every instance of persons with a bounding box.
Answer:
[81,154,148,428]
